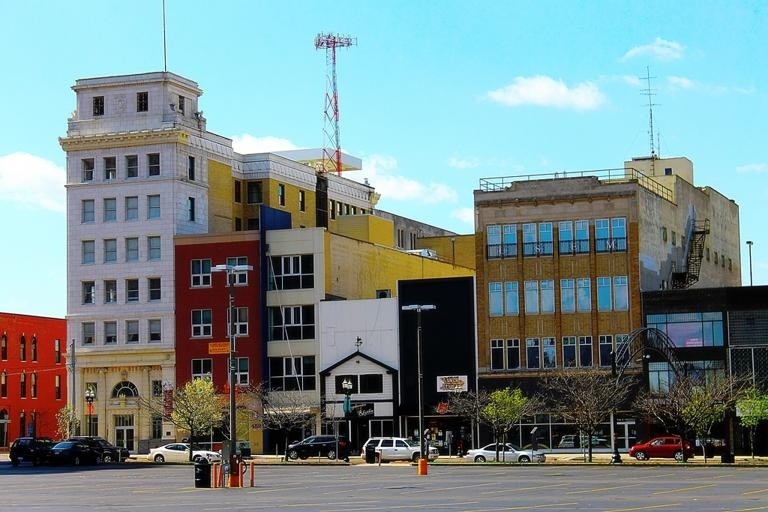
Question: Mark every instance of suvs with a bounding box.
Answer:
[286,433,351,459]
[361,436,439,463]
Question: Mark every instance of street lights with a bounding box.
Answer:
[400,303,438,477]
[342,377,355,456]
[746,240,754,286]
[85,388,96,438]
[209,264,254,487]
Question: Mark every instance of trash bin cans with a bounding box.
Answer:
[704,440,714,458]
[366,444,375,464]
[194,462,213,488]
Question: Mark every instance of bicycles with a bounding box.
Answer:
[219,454,247,475]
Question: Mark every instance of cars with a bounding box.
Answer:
[629,436,695,463]
[465,441,547,465]
[9,435,129,469]
[148,442,221,464]
[558,434,599,448]
